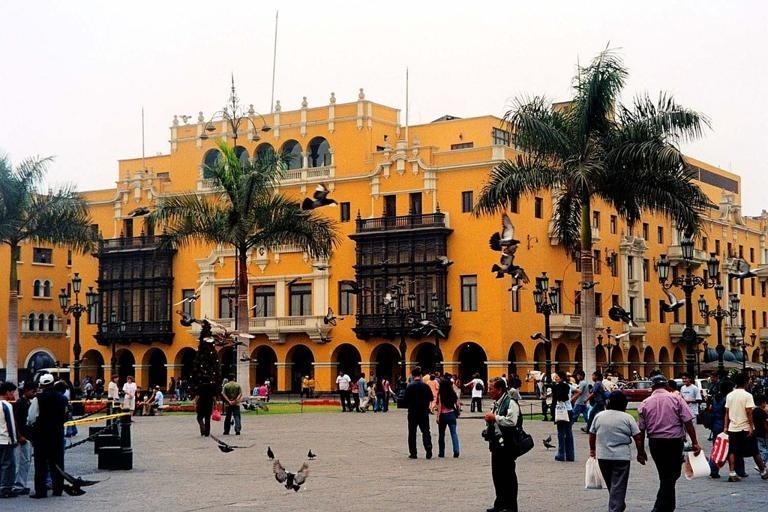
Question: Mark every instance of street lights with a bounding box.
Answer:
[729,324,756,370]
[420,293,451,375]
[382,281,415,409]
[696,279,738,379]
[59,272,96,398]
[655,228,719,385]
[101,310,126,382]
[597,326,619,375]
[532,269,563,383]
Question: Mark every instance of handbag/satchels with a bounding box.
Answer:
[708,432,730,468]
[584,456,608,490]
[505,430,535,460]
[553,401,571,423]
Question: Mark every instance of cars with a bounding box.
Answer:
[614,380,655,402]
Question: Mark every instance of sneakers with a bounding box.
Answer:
[51,488,63,497]
[407,452,418,459]
[487,507,517,512]
[727,471,749,482]
[201,429,210,436]
[453,454,460,459]
[30,488,49,500]
[709,473,721,479]
[754,463,768,479]
[236,432,240,435]
[13,487,31,494]
[0,488,19,498]
[223,431,229,435]
[437,452,445,457]
[425,449,433,459]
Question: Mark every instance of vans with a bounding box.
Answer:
[672,379,708,399]
[34,368,71,388]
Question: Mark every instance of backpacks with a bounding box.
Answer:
[476,382,483,390]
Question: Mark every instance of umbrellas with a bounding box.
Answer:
[703,360,743,367]
[740,362,768,368]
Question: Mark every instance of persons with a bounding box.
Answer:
[484,371,534,512]
[13,382,38,494]
[26,374,66,499]
[300,375,309,398]
[588,390,647,512]
[525,368,767,485]
[436,379,464,458]
[637,376,700,512]
[0,382,18,498]
[309,375,315,398]
[64,371,272,446]
[46,380,73,489]
[402,370,433,460]
[335,372,484,412]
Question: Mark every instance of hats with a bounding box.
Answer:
[38,373,55,386]
[649,373,669,388]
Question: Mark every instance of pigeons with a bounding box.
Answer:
[267,446,317,492]
[420,319,446,338]
[660,288,687,313]
[318,306,337,345]
[242,399,256,411]
[127,206,150,217]
[55,464,111,496]
[437,255,454,269]
[173,279,261,364]
[301,182,338,211]
[728,255,766,280]
[487,211,529,291]
[542,434,555,451]
[605,302,638,341]
[579,280,600,290]
[530,332,551,344]
[210,434,257,453]
